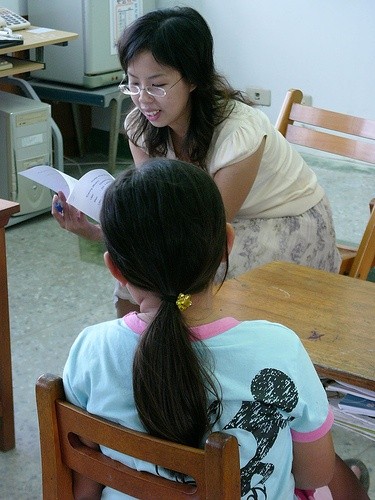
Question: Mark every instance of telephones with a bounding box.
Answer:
[0,7,30,31]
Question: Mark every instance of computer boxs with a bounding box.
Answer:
[0,90,54,217]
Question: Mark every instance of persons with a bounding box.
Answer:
[51,7,342,317]
[61,160,371,498]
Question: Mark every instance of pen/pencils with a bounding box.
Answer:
[55,202,63,213]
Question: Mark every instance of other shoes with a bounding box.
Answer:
[344,458,369,499]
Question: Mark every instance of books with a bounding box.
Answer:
[324,380,375,443]
[0,58,14,71]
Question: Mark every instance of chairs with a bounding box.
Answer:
[274,88,375,279]
[35,373,241,500]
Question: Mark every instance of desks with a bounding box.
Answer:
[0,26,80,79]
[212,260,375,391]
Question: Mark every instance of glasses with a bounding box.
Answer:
[118,74,182,98]
